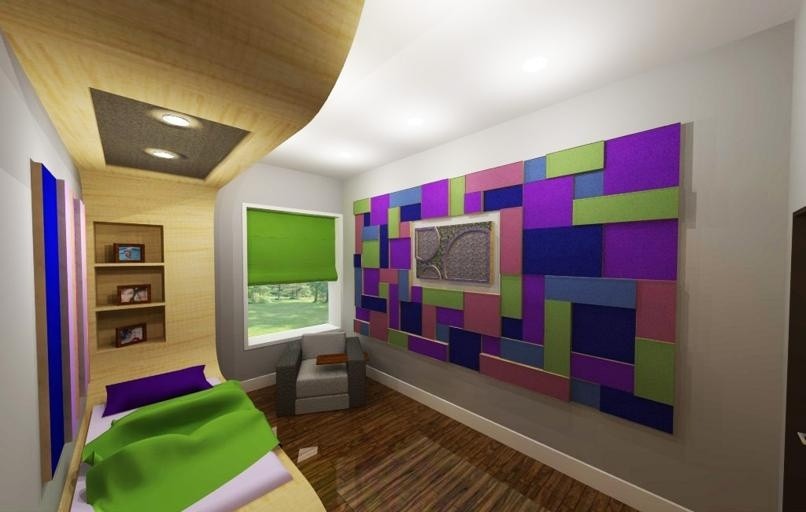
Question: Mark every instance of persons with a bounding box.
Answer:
[136,288,147,303]
[119,288,136,303]
[123,249,131,259]
